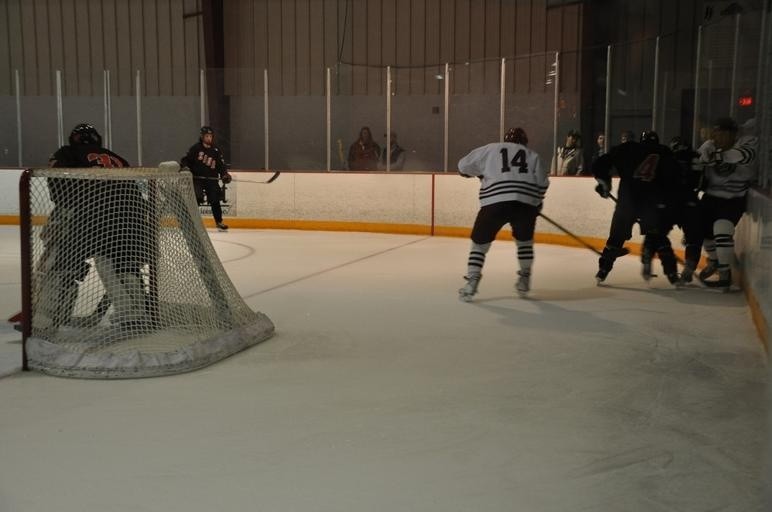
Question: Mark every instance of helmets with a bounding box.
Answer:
[69,123,102,147]
[199,126,214,134]
[640,130,659,143]
[504,127,528,144]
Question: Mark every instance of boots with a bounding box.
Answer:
[457,272,483,296]
[216,221,228,229]
[642,259,732,287]
[595,262,612,282]
[514,268,531,291]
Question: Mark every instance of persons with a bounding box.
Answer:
[348,127,381,171]
[458,128,549,297]
[550,130,586,176]
[589,118,761,288]
[32,123,156,340]
[376,131,405,171]
[180,127,232,230]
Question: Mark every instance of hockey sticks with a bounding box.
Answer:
[61,198,170,327]
[609,194,732,287]
[539,211,630,259]
[193,170,280,185]
